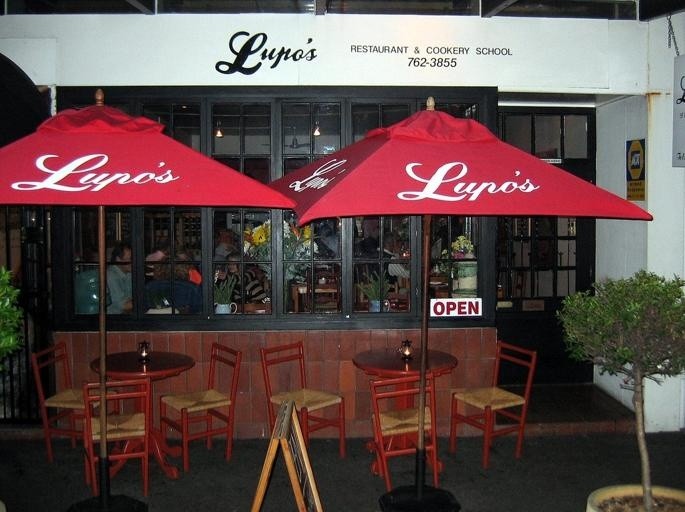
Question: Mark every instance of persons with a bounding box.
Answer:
[285,211,339,269]
[432,216,465,260]
[355,218,410,292]
[214,228,267,303]
[56,240,133,314]
[144,241,201,285]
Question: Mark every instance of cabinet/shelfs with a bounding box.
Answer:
[500,217,595,383]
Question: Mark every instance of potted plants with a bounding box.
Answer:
[213,275,238,315]
[356,268,392,312]
[556,269,684,512]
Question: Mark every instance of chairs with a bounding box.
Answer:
[260,340,347,461]
[450,340,536,471]
[370,373,439,491]
[32,337,242,496]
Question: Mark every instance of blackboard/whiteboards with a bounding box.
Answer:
[280,400,323,512]
